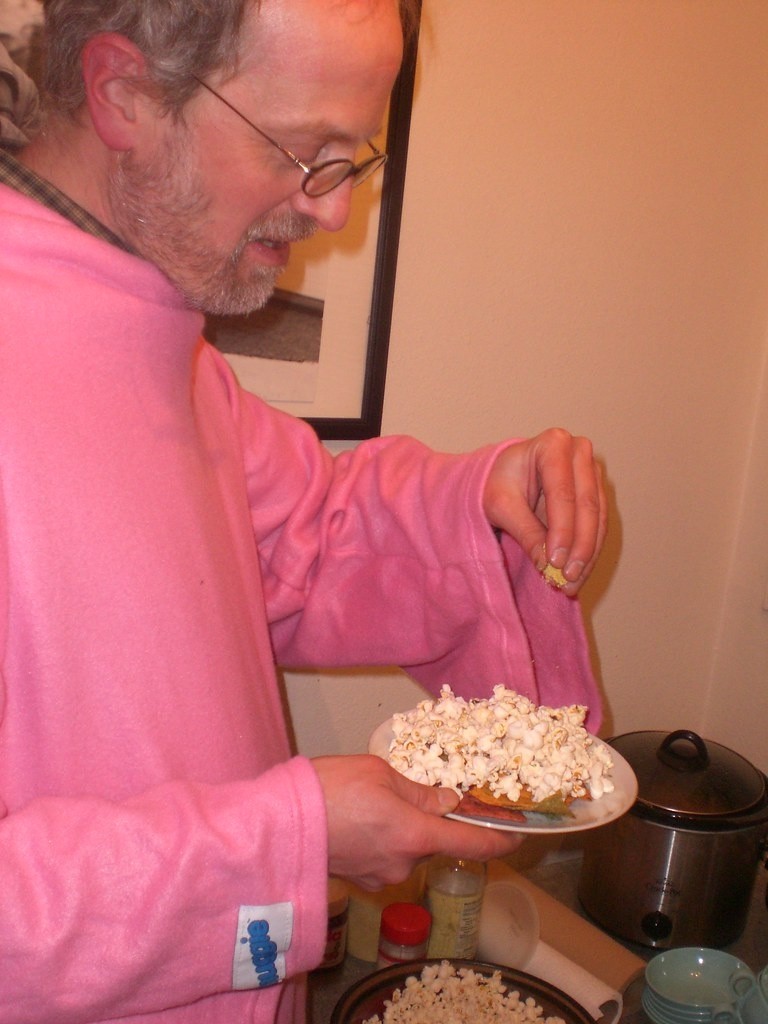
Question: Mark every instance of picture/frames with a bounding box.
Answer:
[202,0,423,443]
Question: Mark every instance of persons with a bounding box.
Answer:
[0,0,610,1024]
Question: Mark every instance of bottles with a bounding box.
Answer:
[424,854,484,960]
[377,903,432,969]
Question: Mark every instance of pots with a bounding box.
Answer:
[578,729,768,947]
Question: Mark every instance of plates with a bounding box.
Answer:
[642,948,754,1024]
[368,711,638,832]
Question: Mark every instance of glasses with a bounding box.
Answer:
[188,70,388,197]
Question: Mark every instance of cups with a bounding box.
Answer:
[709,964,768,1024]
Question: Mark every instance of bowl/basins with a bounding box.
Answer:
[333,960,595,1024]
